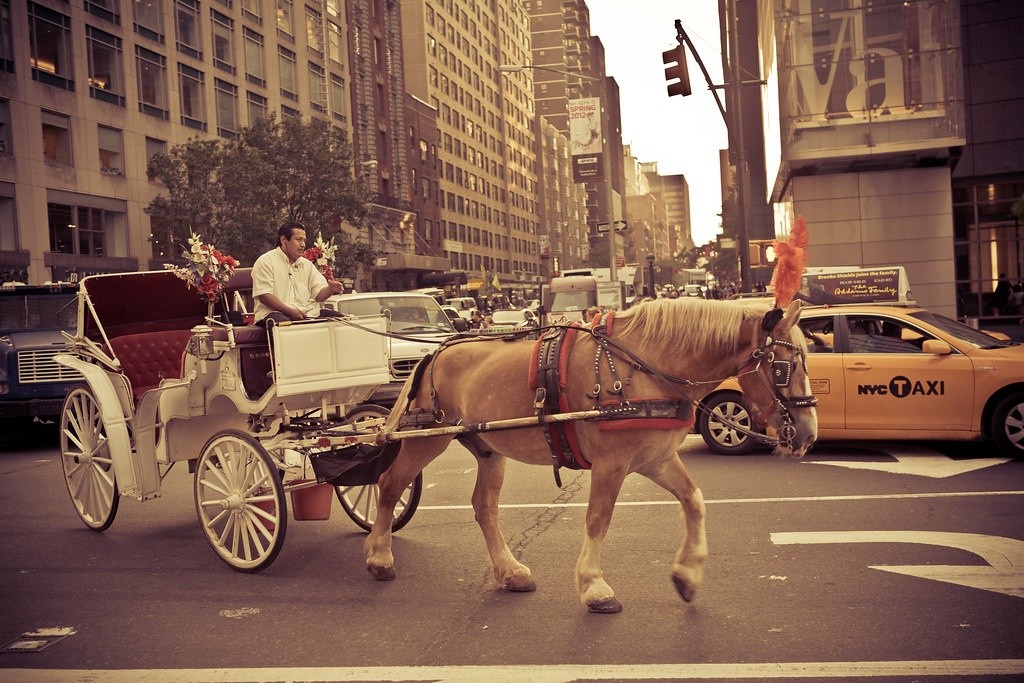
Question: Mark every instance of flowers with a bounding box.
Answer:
[298,230,341,282]
[162,226,240,304]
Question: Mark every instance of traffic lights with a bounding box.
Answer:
[764,242,778,267]
[662,45,692,97]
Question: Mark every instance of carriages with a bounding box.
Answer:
[52,214,819,614]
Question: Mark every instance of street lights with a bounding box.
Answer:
[645,252,657,299]
[500,65,618,281]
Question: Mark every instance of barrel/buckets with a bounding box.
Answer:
[247,489,276,533]
[286,479,333,521]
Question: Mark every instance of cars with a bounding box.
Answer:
[324,290,469,405]
[688,266,1024,460]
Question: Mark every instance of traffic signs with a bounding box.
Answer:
[596,220,628,234]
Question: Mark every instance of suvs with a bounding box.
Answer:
[489,307,540,329]
[1,281,92,444]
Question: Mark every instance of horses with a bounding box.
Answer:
[362,298,818,614]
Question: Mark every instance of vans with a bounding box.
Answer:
[440,297,478,333]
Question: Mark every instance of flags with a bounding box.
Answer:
[492,274,501,291]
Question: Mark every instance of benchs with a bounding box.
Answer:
[208,324,275,399]
[103,329,194,434]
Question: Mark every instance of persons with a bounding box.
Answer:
[992,273,1016,321]
[251,222,344,329]
[794,275,872,305]
[484,295,519,309]
[670,289,680,298]
[467,309,492,329]
[757,281,766,296]
[705,282,739,299]
